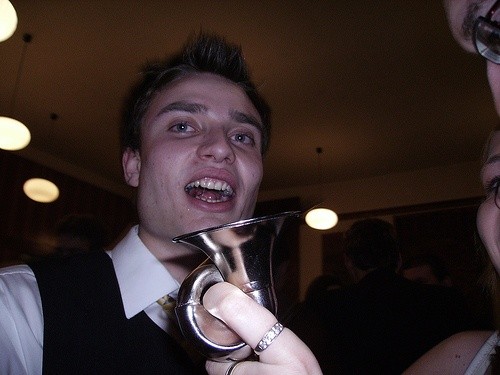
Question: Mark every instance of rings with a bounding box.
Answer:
[254,322,284,352]
[224,359,246,374]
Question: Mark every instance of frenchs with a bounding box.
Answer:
[171,210,304,362]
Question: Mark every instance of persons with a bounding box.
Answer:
[272,0,500,374]
[0,31,324,375]
[52,213,105,258]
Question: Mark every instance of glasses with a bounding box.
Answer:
[472,0,500,65]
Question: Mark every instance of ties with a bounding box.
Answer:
[156,294,179,339]
